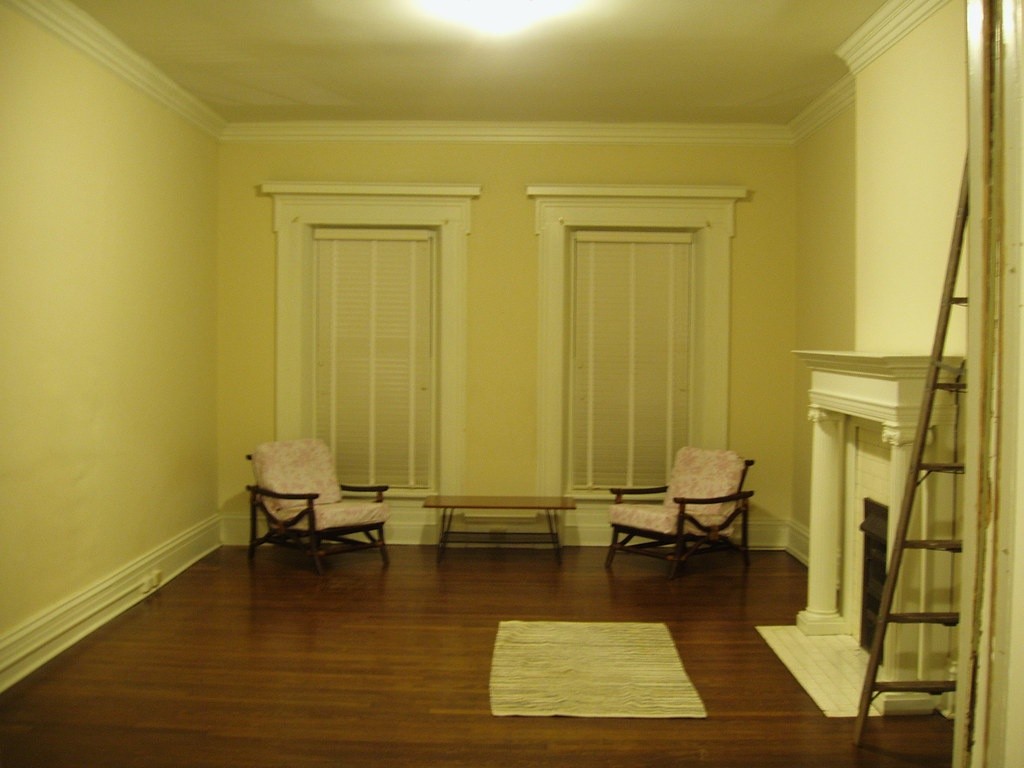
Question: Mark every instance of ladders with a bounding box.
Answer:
[852,146,969,743]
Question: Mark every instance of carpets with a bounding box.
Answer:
[491,620,707,720]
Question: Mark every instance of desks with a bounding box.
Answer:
[423,495,578,566]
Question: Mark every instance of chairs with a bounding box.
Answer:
[246,437,391,574]
[606,446,755,581]
